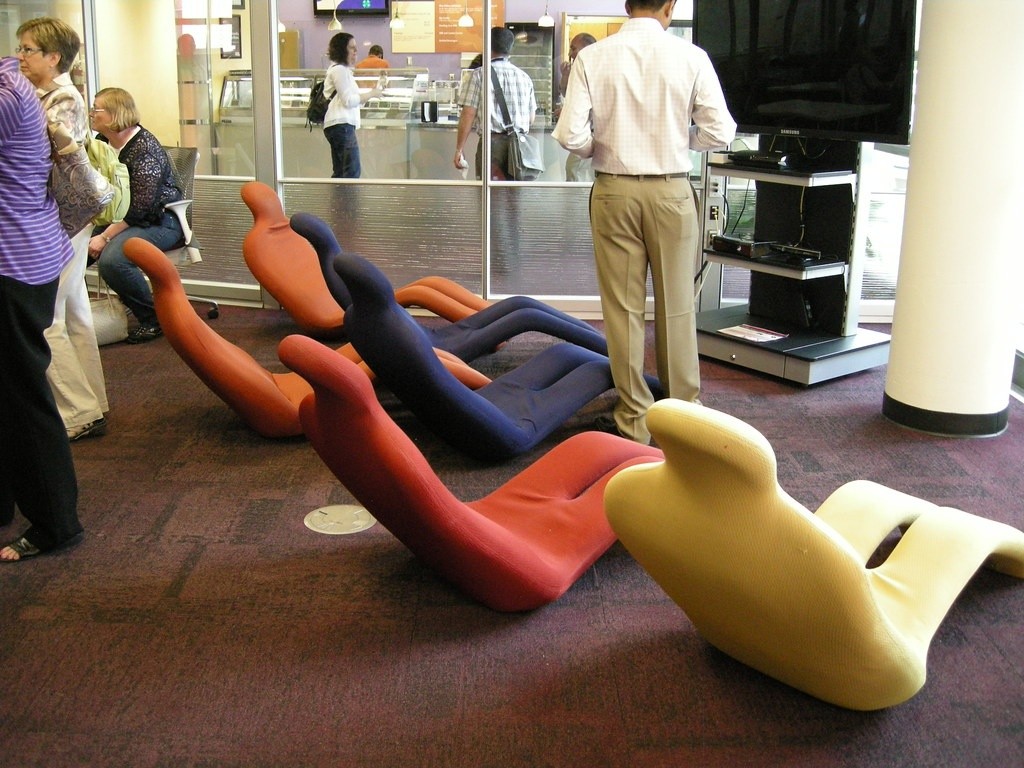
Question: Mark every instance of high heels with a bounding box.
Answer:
[65,416,108,443]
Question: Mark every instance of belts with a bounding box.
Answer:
[594,169,689,181]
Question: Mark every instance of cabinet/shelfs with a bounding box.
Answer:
[696,129,891,390]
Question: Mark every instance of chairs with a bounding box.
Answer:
[278,332,665,614]
[288,211,610,364]
[120,236,493,441]
[243,180,495,338]
[605,399,1024,710]
[332,251,660,464]
[96,146,221,318]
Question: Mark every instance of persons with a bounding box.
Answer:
[559,34,599,257]
[549,0,741,447]
[354,44,390,89]
[0,54,89,562]
[454,26,538,279]
[176,33,211,151]
[15,16,114,440]
[322,32,385,244]
[84,87,183,344]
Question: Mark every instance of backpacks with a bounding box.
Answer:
[44,93,129,225]
[304,63,343,132]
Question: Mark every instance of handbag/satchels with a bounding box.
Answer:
[506,132,544,181]
[45,121,115,240]
[87,258,129,347]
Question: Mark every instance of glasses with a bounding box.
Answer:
[15,47,44,57]
[90,108,106,113]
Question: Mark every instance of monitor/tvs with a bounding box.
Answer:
[695,0,917,144]
[313,0,390,15]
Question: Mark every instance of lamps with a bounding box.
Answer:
[457,0,474,28]
[389,0,404,29]
[327,0,342,31]
[537,0,554,28]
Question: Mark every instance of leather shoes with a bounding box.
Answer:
[595,415,623,437]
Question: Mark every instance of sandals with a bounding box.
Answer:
[0,524,85,563]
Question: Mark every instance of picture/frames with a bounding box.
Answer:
[231,0,245,9]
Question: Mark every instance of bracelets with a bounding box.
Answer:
[101,233,111,243]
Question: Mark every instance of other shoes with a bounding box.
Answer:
[126,325,162,344]
